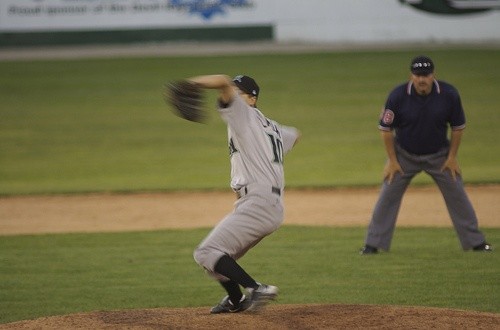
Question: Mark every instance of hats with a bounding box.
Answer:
[410,56,435,75]
[231,72,261,98]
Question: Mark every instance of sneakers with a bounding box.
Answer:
[360,244,380,255]
[470,244,491,251]
[239,282,278,314]
[210,297,248,313]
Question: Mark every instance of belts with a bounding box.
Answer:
[236,185,281,198]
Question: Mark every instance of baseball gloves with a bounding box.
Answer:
[168,79,207,123]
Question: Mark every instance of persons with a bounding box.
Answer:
[185,75,301,315]
[358,56,491,255]
